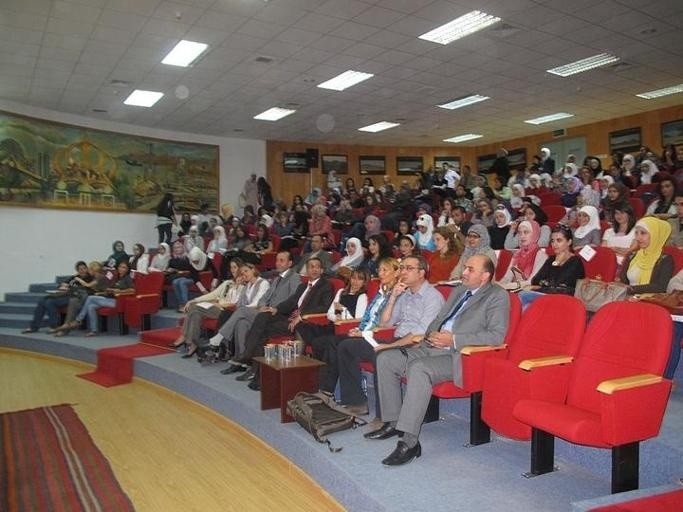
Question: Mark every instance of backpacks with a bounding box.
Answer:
[286,391,367,452]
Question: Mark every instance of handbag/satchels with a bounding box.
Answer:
[503,266,527,293]
[572,276,626,312]
[640,289,683,315]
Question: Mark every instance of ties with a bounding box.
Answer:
[265,277,281,306]
[437,291,471,332]
[288,284,311,321]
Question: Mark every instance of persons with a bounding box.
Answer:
[22,146,681,470]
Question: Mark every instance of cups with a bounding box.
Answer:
[263,341,302,364]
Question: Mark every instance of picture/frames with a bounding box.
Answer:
[607,126,642,157]
[660,119,683,147]
[0,109,221,216]
[282,147,528,174]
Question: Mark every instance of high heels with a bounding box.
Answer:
[181,347,197,357]
[167,340,186,348]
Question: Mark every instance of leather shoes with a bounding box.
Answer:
[228,358,252,368]
[381,441,421,465]
[220,364,246,374]
[191,336,218,352]
[364,423,403,439]
[236,370,255,380]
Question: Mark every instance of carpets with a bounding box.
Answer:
[0,398,135,512]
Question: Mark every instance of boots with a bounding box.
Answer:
[221,343,231,360]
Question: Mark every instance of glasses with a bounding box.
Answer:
[467,234,479,239]
[400,265,422,271]
[555,224,569,231]
[232,220,238,223]
[493,206,506,210]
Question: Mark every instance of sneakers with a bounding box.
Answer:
[56,331,69,335]
[338,401,368,415]
[68,321,80,328]
[365,417,385,431]
[85,331,96,336]
[22,329,36,333]
[177,307,185,312]
